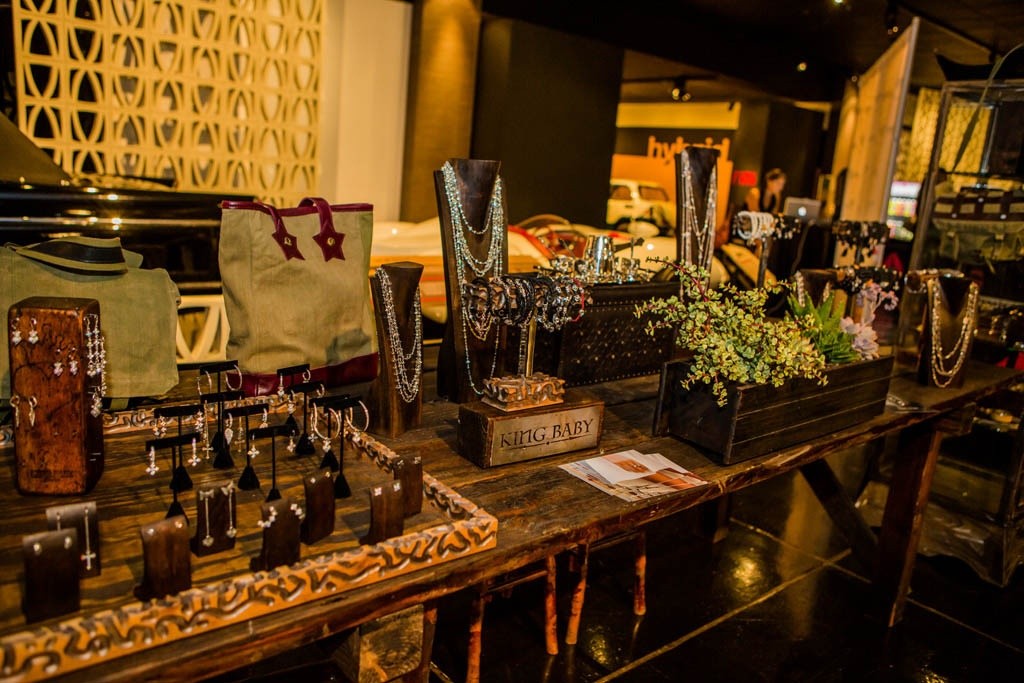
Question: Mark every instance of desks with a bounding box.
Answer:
[0,328,1024,683]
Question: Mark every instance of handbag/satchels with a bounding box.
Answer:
[1,237,179,410]
[219,197,379,396]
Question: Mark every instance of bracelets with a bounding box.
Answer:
[904,269,937,293]
[733,212,800,247]
[458,266,589,341]
[829,264,900,296]
[831,219,887,257]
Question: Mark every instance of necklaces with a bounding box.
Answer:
[377,262,428,406]
[923,273,979,388]
[440,159,506,395]
[677,145,719,297]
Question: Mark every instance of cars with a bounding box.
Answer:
[605,178,677,238]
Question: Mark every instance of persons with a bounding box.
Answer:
[740,169,787,212]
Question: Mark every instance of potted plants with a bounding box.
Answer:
[633,245,899,465]
